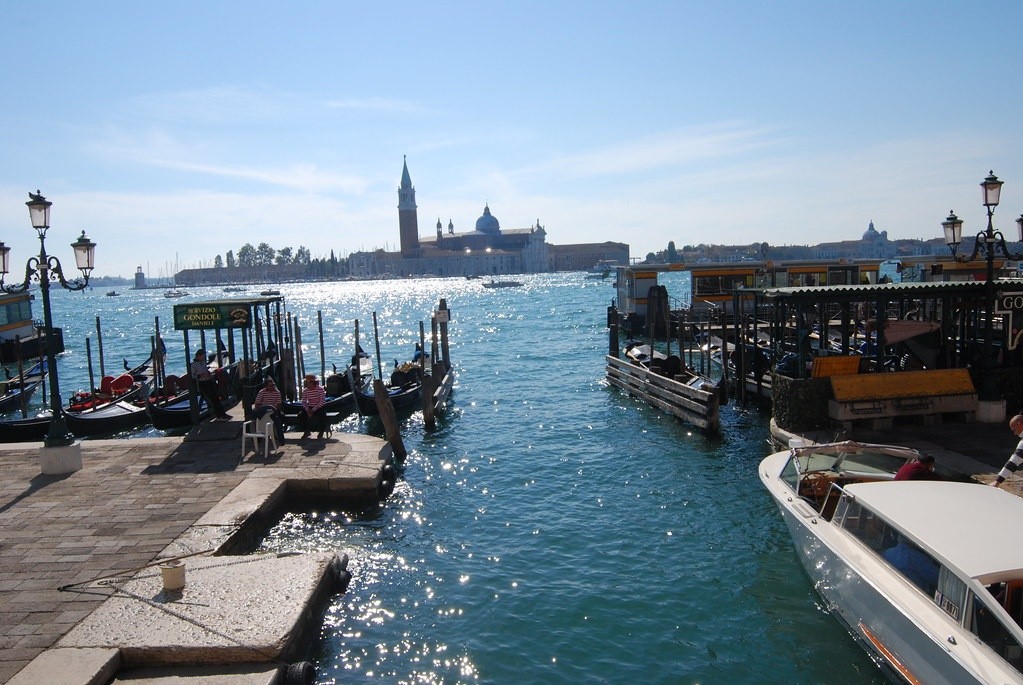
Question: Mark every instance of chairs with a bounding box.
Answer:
[241,405,277,457]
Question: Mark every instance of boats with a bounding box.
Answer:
[0,290,66,367]
[260,288,281,296]
[757,429,1023,685]
[222,284,249,293]
[283,341,374,424]
[163,287,190,299]
[0,353,50,418]
[60,328,284,439]
[481,279,525,289]
[618,313,898,409]
[105,290,122,297]
[346,360,433,417]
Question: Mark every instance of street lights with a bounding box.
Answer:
[940,170,1023,370]
[0,188,98,450]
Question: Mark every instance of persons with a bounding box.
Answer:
[299,373,326,440]
[992,412,1023,485]
[254,377,284,446]
[192,349,234,422]
[881,532,938,598]
[893,454,934,479]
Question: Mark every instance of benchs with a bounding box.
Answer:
[133,381,145,386]
[281,411,341,439]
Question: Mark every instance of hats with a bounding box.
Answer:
[303,374,319,386]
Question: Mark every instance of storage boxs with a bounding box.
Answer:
[975,399,1007,423]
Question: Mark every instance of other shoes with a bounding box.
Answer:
[217,414,235,421]
[316,432,324,440]
[281,440,286,446]
[301,431,313,440]
